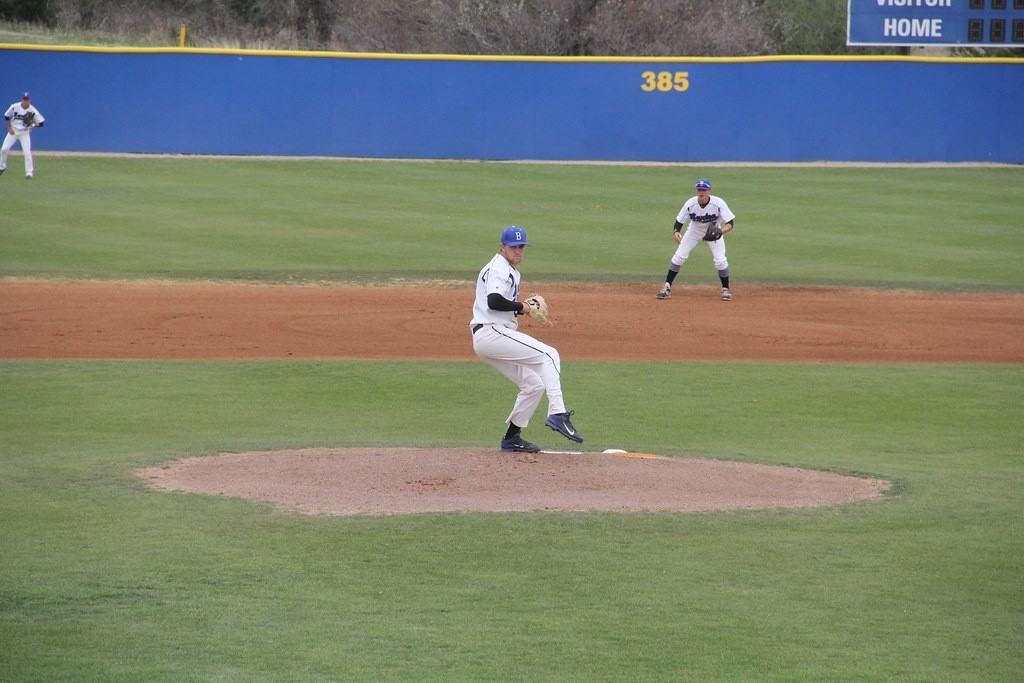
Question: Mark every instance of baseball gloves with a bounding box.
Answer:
[23,111,35,126]
[702,221,722,242]
[524,292,548,325]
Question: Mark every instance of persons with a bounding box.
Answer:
[657,177,736,301]
[0,92,45,179]
[469,227,584,452]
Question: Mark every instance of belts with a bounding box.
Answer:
[473,323,483,334]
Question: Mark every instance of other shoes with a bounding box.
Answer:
[0,168,6,176]
[25,172,32,179]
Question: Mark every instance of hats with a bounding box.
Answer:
[501,226,532,247]
[21,91,32,101]
[695,178,711,190]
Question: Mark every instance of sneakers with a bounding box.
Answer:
[500,430,540,453]
[721,288,731,300]
[656,285,672,299]
[545,408,584,443]
